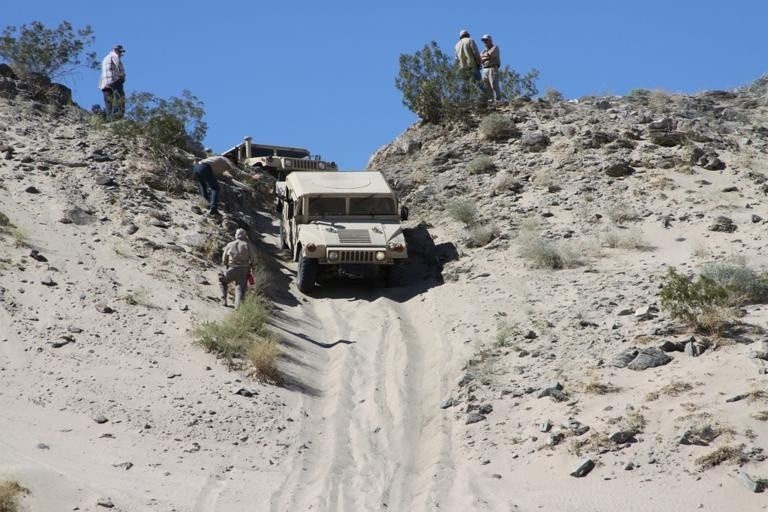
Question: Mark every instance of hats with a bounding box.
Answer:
[115,44,125,52]
[459,29,471,38]
[481,35,492,40]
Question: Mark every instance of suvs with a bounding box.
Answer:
[275,171,412,290]
[217,136,339,183]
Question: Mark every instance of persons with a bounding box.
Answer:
[267,185,292,203]
[455,31,484,109]
[194,153,261,216]
[218,228,256,311]
[99,45,125,122]
[480,34,501,102]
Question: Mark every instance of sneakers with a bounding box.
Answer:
[210,207,220,214]
